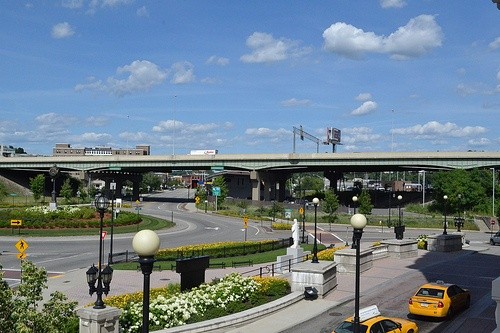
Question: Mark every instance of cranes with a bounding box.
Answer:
[293,126,341,153]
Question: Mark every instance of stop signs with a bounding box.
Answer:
[491,220,495,225]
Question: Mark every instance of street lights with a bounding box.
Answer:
[85,195,113,309]
[132,229,160,333]
[458,194,461,232]
[490,168,494,230]
[311,198,320,264]
[349,213,367,333]
[352,196,358,249]
[397,195,403,239]
[443,196,448,235]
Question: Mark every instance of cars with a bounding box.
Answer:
[490,229,500,246]
[409,284,471,320]
[334,315,418,333]
